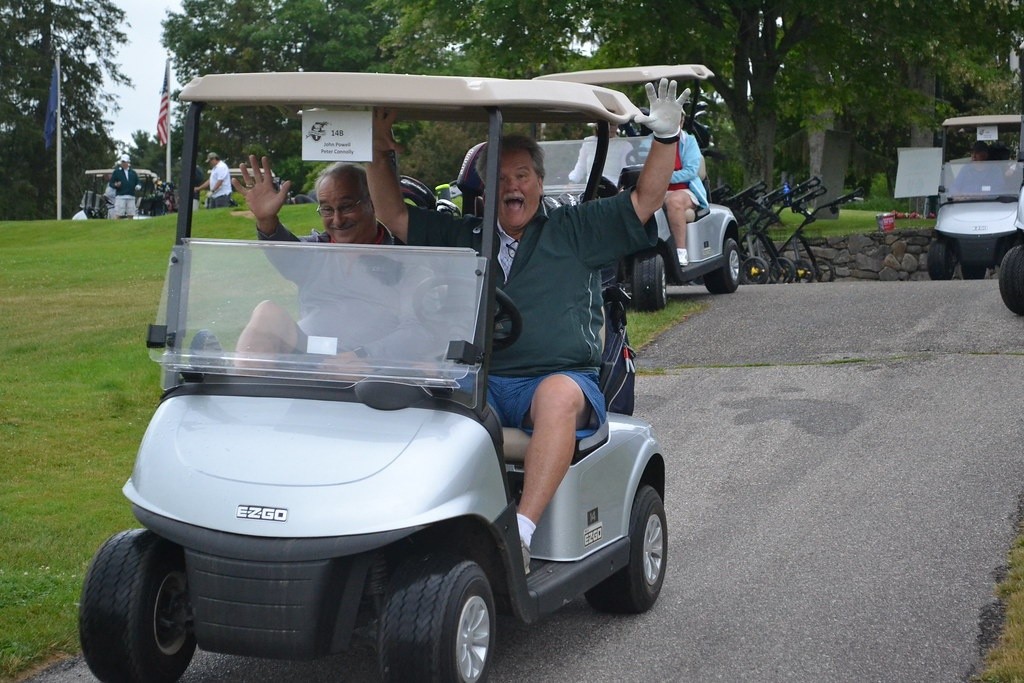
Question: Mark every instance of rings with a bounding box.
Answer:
[245,182,254,188]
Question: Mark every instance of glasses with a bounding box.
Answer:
[316,192,364,217]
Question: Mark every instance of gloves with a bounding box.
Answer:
[205,191,213,198]
[134,185,142,191]
[634,78,692,140]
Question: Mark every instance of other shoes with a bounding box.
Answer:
[190,327,229,377]
[520,537,532,576]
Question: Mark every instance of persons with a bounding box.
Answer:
[192,77,710,576]
[109,153,141,221]
[946,141,1006,203]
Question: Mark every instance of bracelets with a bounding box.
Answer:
[654,132,680,144]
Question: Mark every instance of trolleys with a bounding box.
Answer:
[718,173,865,283]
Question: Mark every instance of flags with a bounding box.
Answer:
[157,63,169,147]
[42,62,59,150]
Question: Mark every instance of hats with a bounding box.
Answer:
[965,141,990,152]
[204,151,220,163]
[120,154,131,163]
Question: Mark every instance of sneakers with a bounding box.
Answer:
[676,247,688,266]
